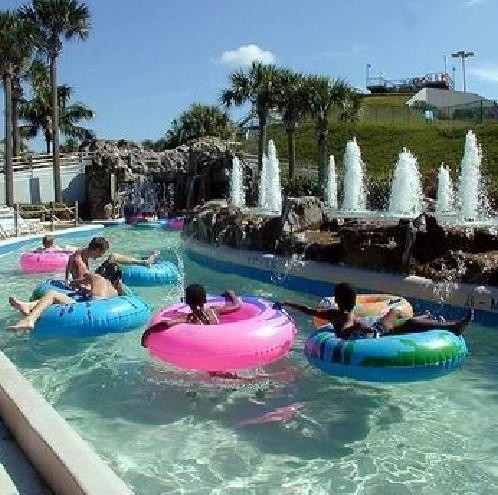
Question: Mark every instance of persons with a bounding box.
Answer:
[138,203,186,222]
[140,283,241,348]
[271,281,475,341]
[102,199,119,220]
[5,235,161,333]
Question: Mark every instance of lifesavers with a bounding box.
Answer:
[115,256,179,284]
[147,297,295,369]
[304,319,469,382]
[129,213,184,228]
[20,247,75,272]
[24,279,151,337]
[315,292,413,327]
